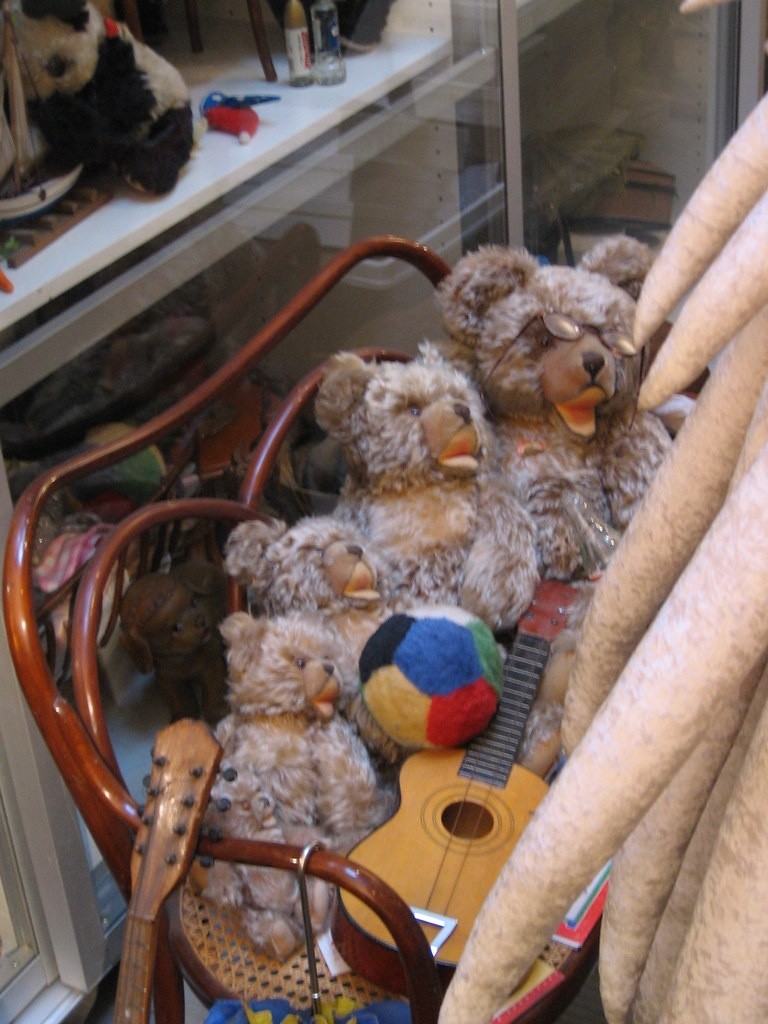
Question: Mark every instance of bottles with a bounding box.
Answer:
[310,0,346,86]
[285,0,315,87]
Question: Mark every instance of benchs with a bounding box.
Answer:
[3,235,711,1024]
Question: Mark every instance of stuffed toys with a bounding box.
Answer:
[0,0,194,195]
[194,91,279,144]
[119,234,696,961]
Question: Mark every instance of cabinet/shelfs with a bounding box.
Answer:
[0,1,733,1024]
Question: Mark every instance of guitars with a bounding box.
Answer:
[329,575,571,994]
